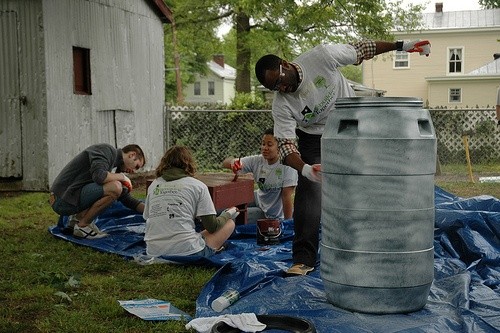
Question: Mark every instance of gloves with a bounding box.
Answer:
[301,164,322,183]
[396,39,430,57]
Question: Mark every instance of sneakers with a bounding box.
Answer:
[67,214,80,227]
[73,223,102,239]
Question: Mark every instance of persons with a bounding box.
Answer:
[221,129,298,226]
[49,144,146,241]
[255,40,431,276]
[142,145,240,259]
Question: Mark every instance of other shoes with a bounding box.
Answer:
[287,264,314,276]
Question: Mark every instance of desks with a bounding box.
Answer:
[144,175,256,225]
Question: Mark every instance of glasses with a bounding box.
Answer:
[271,65,286,91]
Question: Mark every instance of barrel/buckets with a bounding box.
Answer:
[319,96,434,316]
[256,216,282,246]
[210,315,317,333]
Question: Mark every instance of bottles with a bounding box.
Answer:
[211,288,240,313]
